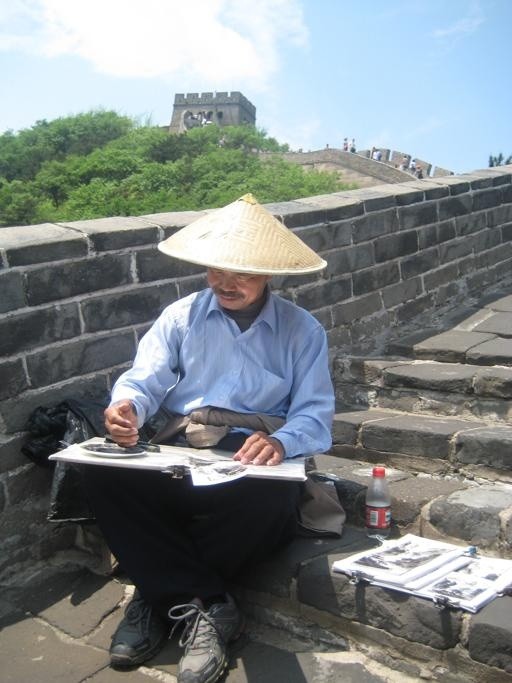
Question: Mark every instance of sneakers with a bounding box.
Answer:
[108,583,180,667]
[177,588,247,683]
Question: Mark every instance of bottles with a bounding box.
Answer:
[364,467,392,540]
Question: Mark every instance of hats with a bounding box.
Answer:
[156,192,328,276]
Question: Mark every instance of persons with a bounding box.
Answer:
[343,135,424,179]
[88,267,335,683]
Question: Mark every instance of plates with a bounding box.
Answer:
[77,441,146,460]
